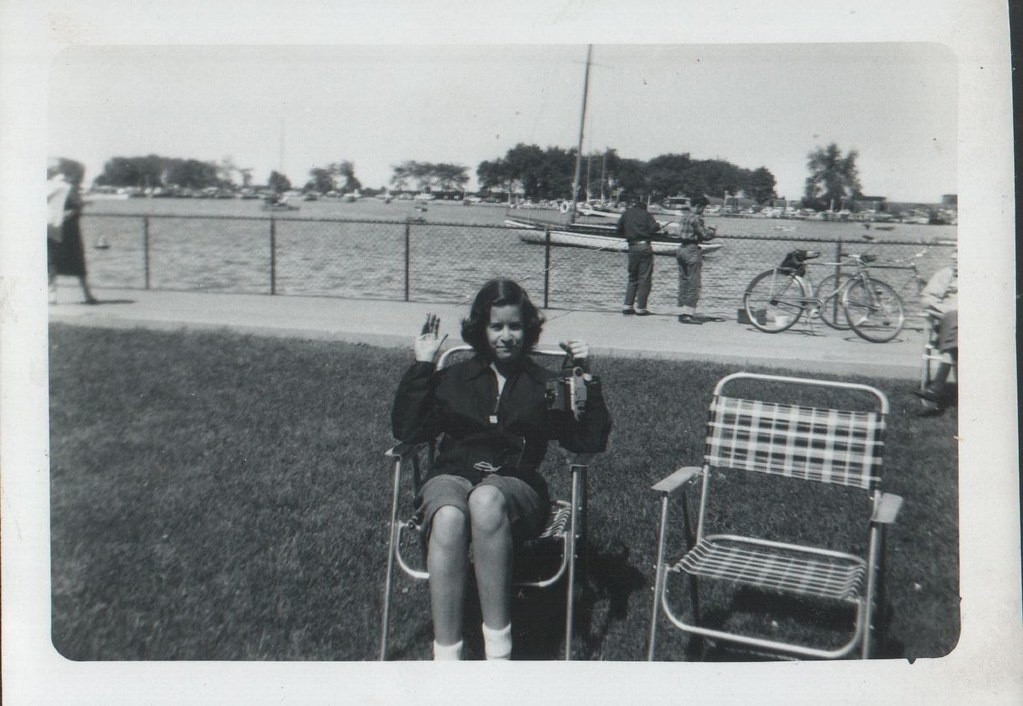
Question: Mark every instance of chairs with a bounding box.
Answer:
[916,312,958,390]
[379,345,588,660]
[647,373,904,662]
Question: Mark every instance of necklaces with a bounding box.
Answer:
[47,158,96,303]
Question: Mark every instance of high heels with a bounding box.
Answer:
[914,387,945,410]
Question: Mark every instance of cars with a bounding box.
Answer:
[804,209,815,215]
[463,195,482,202]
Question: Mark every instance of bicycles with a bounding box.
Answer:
[813,244,927,331]
[743,235,905,344]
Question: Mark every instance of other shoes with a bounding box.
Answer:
[678,313,701,324]
[636,311,655,316]
[623,310,637,314]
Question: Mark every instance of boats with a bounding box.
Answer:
[847,213,880,222]
[258,205,299,212]
[414,193,435,200]
[414,207,427,212]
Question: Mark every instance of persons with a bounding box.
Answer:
[911,247,957,401]
[619,193,662,317]
[391,276,613,661]
[676,195,716,325]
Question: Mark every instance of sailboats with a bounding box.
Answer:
[502,44,724,256]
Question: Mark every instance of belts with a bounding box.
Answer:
[629,241,650,245]
